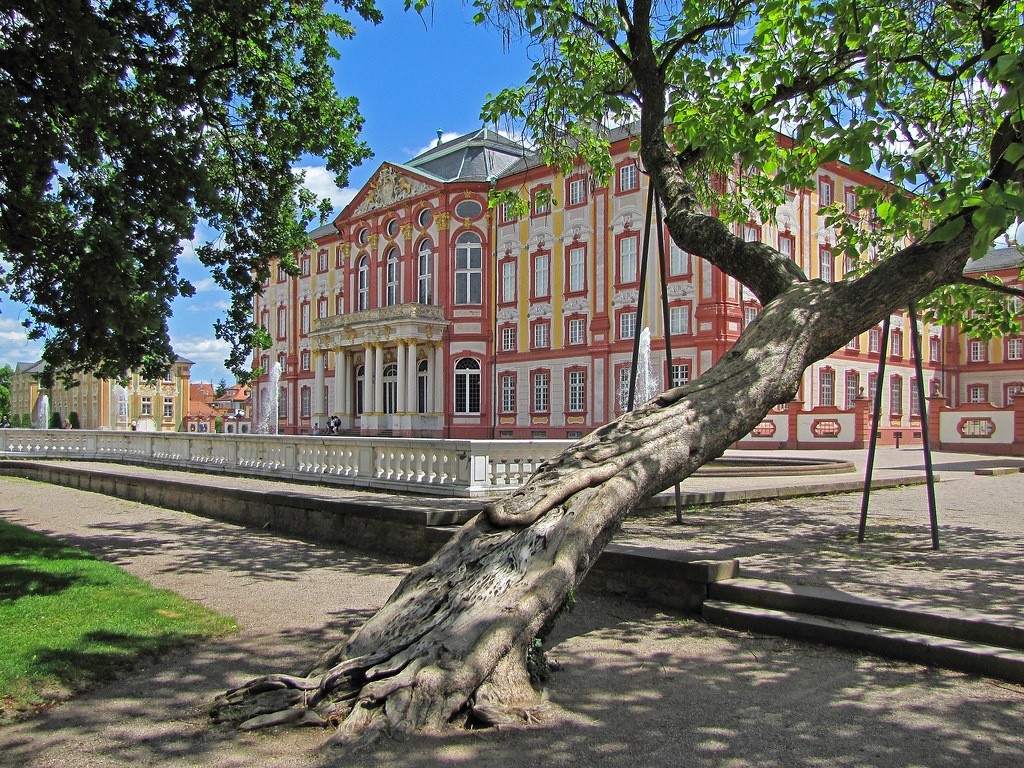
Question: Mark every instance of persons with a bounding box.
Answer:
[324,416,341,435]
[312,422,318,436]
[65,419,72,429]
[0,416,10,428]
[130,418,137,431]
[199,421,204,433]
